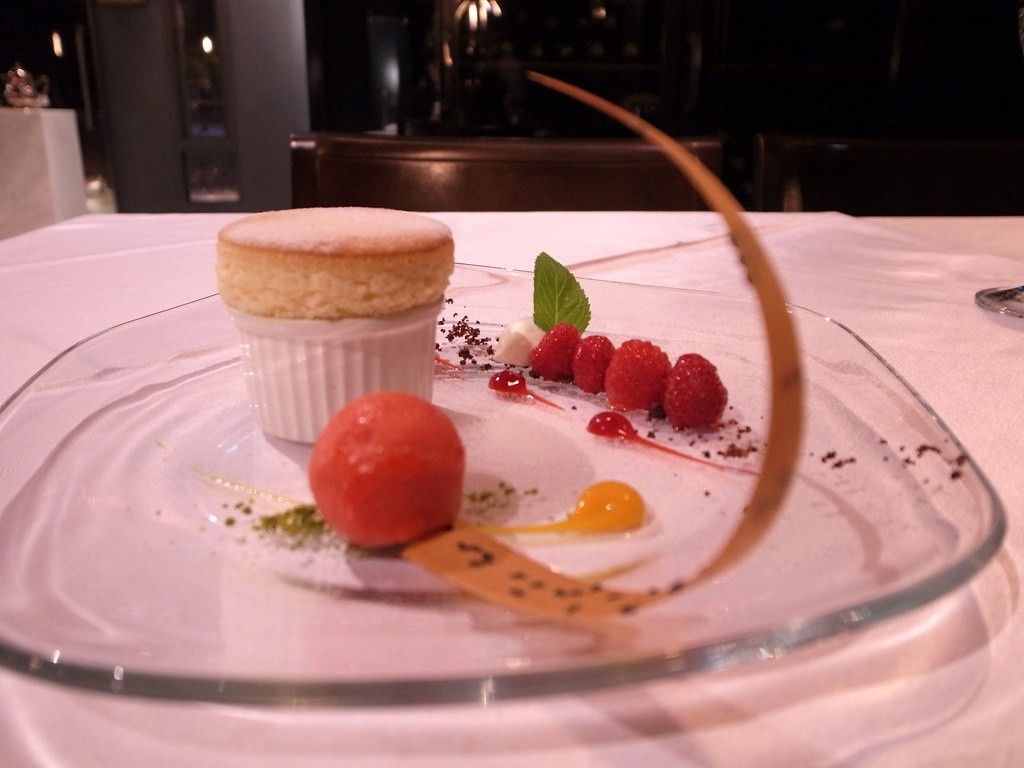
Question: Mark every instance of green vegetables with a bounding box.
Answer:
[532,251,591,335]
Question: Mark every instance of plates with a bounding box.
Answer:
[0,262,1011,707]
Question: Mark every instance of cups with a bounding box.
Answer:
[231,296,448,444]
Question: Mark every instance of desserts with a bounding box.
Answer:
[308,75,806,621]
[216,204,454,442]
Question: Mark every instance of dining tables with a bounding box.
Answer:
[0,212,1024,768]
